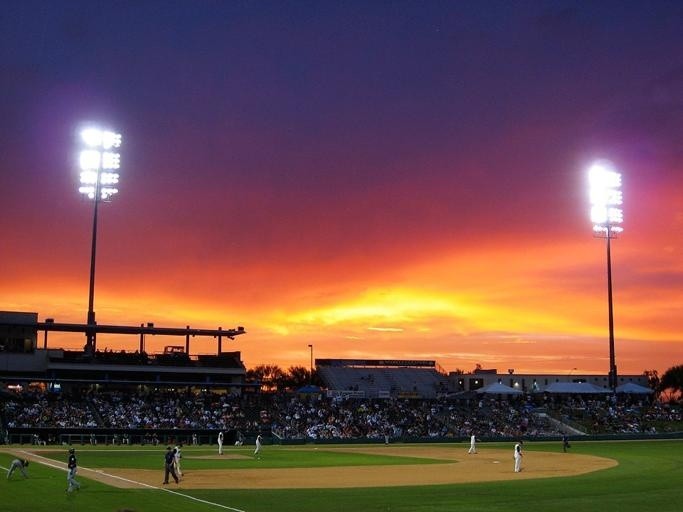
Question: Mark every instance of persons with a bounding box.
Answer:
[512,440,524,472]
[5,457,29,481]
[216,430,225,455]
[561,435,570,452]
[172,442,184,477]
[0,378,683,445]
[65,448,80,493]
[253,430,263,456]
[160,445,178,485]
[467,431,479,454]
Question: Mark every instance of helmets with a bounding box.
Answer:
[24,461,29,466]
[69,448,75,454]
[167,446,171,450]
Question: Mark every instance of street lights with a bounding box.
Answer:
[567,367,578,376]
[586,164,624,391]
[74,127,124,354]
[307,344,313,385]
